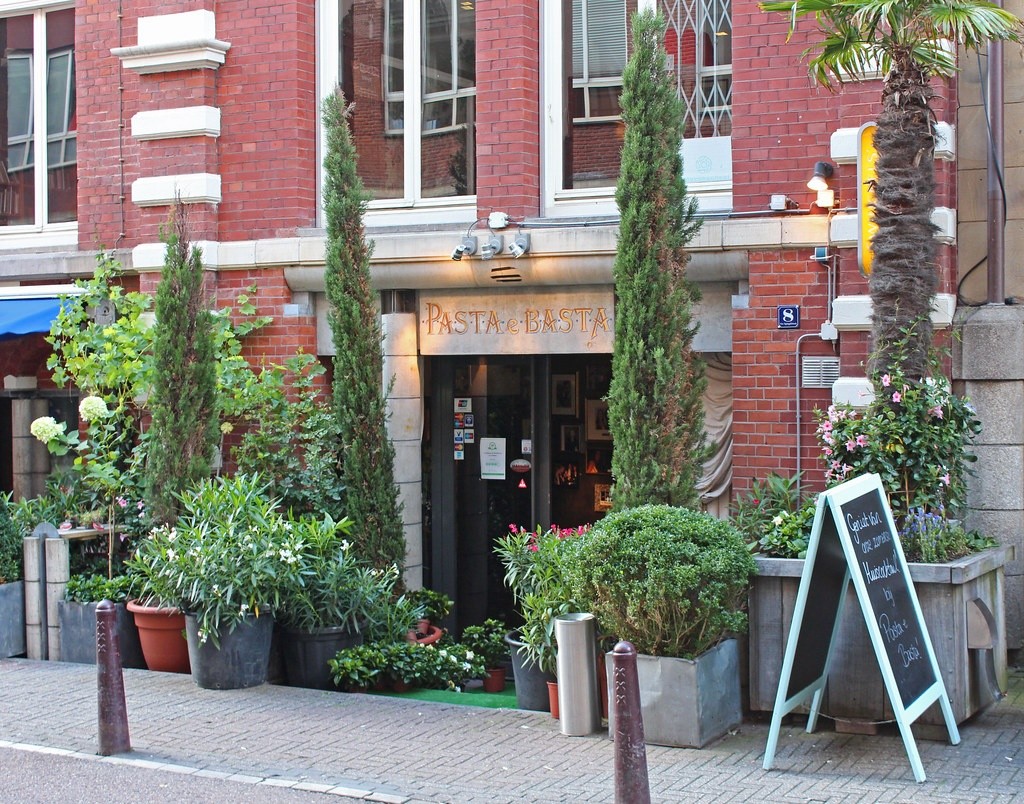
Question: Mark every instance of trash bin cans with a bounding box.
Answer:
[554,612,602,737]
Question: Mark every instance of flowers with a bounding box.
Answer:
[728,375,993,562]
[493,521,594,641]
[116,495,178,608]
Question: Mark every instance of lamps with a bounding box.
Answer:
[451,212,532,261]
[806,162,833,191]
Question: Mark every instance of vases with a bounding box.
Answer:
[504,626,559,712]
[126,596,192,675]
[748,540,1016,741]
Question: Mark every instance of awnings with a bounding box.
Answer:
[0,283,92,335]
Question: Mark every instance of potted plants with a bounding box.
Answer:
[271,510,508,699]
[121,474,317,690]
[0,495,30,659]
[515,585,586,719]
[81,510,99,529]
[56,573,149,669]
[563,507,748,748]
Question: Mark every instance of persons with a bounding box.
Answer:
[597,409,607,430]
[565,430,579,452]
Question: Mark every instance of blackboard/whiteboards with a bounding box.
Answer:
[826,472,942,726]
